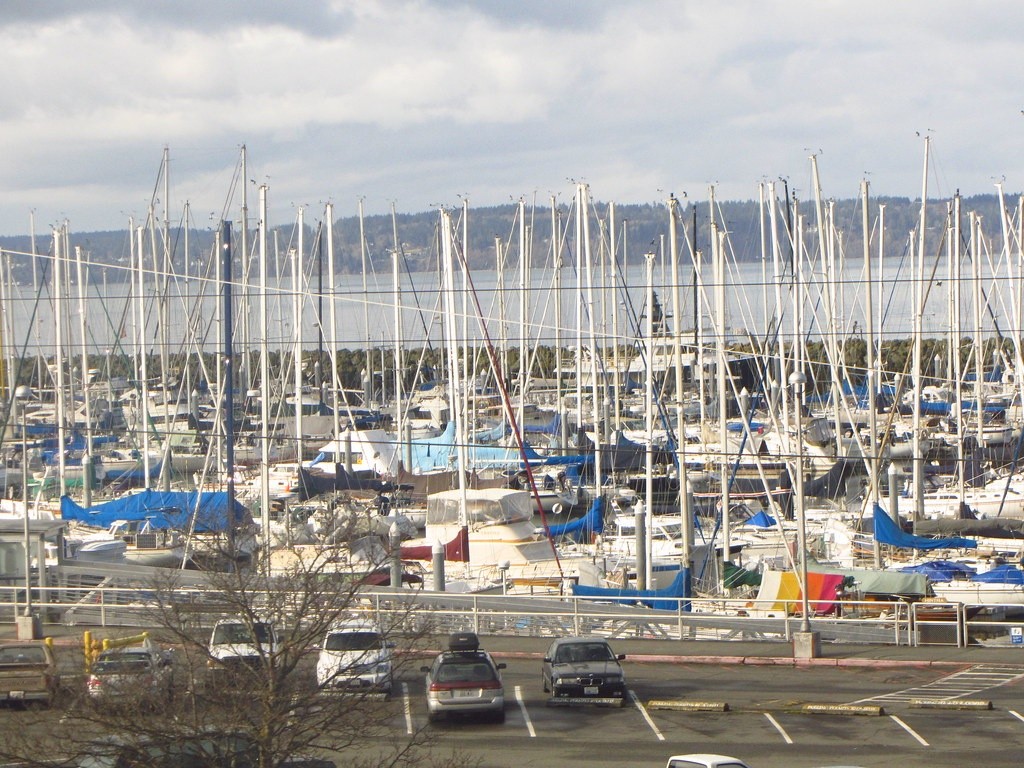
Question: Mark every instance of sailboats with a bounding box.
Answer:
[0,135,1023,611]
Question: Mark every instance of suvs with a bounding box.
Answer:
[420,632,507,721]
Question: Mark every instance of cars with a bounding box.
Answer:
[666,754,750,768]
[0,643,61,708]
[312,628,398,693]
[86,647,173,714]
[541,637,627,707]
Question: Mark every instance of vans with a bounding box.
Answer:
[201,618,285,690]
